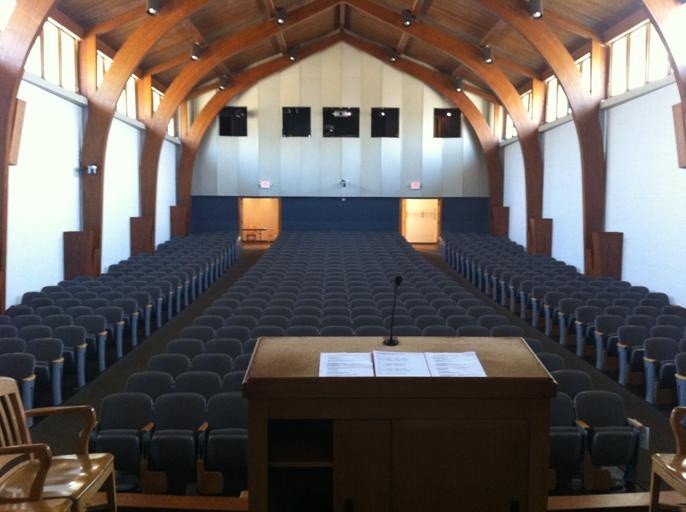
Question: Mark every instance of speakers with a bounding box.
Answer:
[283,107,311,136]
[434,108,461,138]
[219,107,247,136]
[323,108,359,137]
[371,108,399,136]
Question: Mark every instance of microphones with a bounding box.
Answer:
[383,276,402,346]
